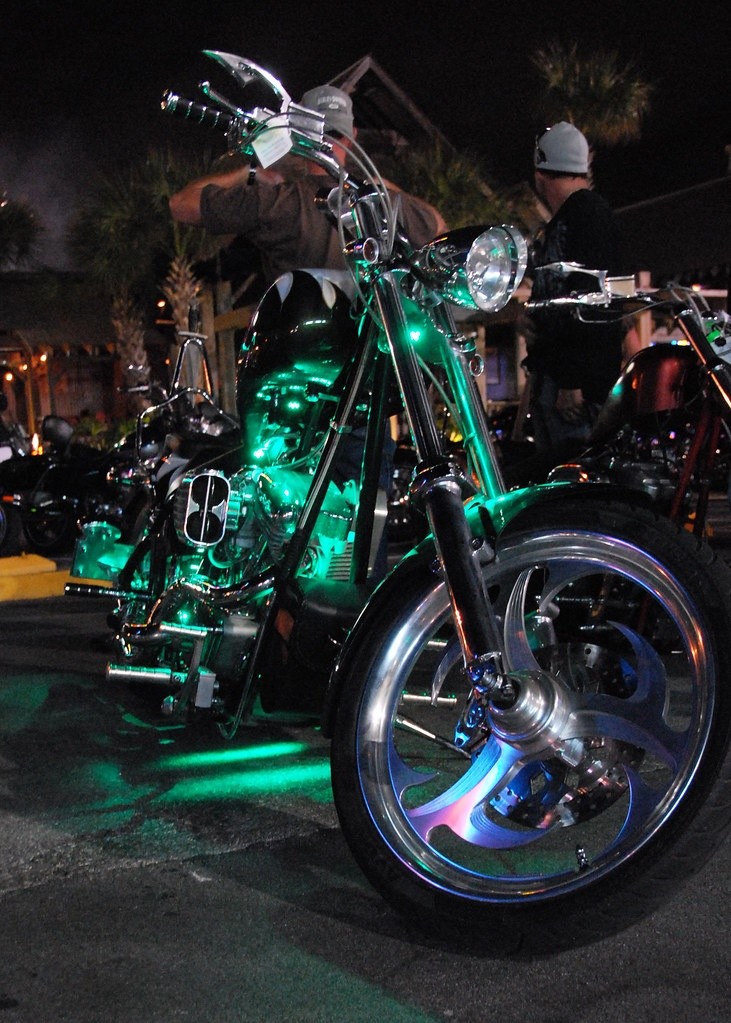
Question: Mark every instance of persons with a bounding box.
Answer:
[71,410,119,441]
[169,84,444,725]
[520,122,640,600]
[654,313,685,344]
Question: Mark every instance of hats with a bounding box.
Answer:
[302,86,354,136]
[534,121,589,173]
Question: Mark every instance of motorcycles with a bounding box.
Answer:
[0,262,730,557]
[64,49,731,940]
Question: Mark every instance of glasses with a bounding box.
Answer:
[536,127,552,163]
